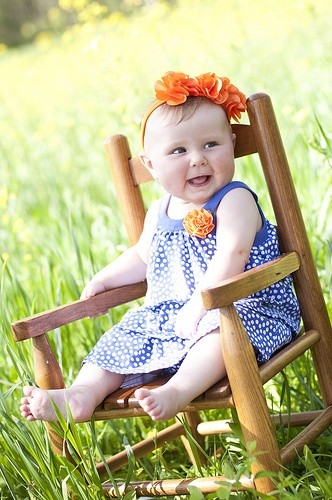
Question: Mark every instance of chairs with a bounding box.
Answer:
[10,92,332,500]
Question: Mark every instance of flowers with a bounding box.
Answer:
[182,208,214,238]
[156,71,247,121]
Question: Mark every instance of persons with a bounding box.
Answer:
[20,70,306,424]
